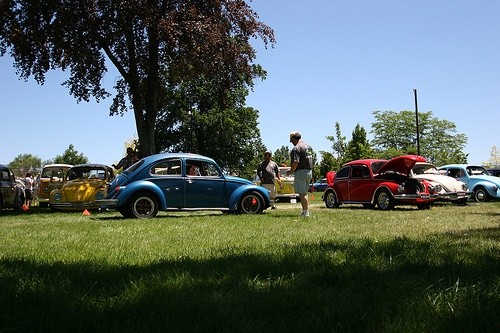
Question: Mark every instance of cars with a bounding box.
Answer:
[321,156,439,211]
[94,154,272,218]
[253,167,301,203]
[308,178,327,191]
[0,164,26,215]
[47,165,115,212]
[37,164,76,209]
[409,162,474,206]
[436,164,500,203]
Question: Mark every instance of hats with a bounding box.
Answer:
[290,132,301,142]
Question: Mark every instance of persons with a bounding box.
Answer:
[286,132,312,216]
[257,151,281,210]
[111,147,133,171]
[32,172,40,207]
[186,164,196,175]
[17,173,33,208]
[130,151,139,164]
